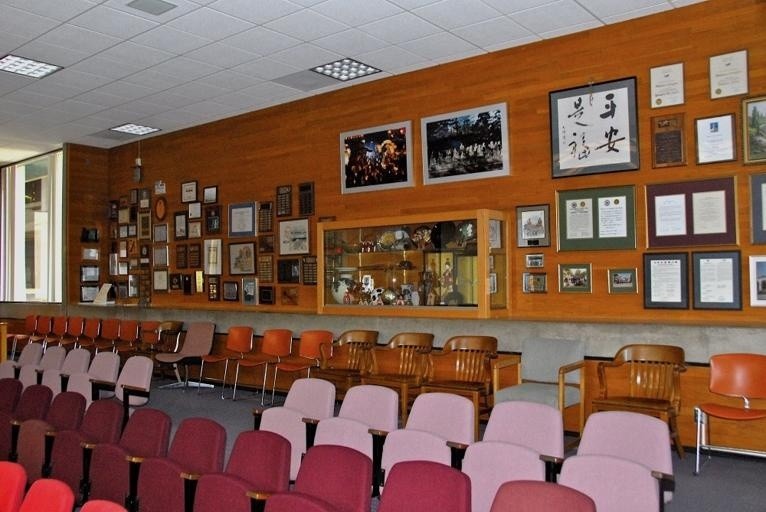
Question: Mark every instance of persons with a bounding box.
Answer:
[345,139,407,187]
[431,141,503,165]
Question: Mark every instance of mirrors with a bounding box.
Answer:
[0,148,63,305]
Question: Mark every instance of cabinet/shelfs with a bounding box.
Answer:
[317,209,515,311]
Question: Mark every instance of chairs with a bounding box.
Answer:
[372,332,436,424]
[196,326,259,400]
[1,314,185,508]
[493,334,586,433]
[156,322,218,392]
[269,331,334,404]
[695,352,766,479]
[188,377,677,509]
[593,343,688,464]
[423,336,503,440]
[230,328,294,401]
[306,329,383,402]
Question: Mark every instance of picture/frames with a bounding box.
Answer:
[515,204,551,246]
[707,47,751,100]
[741,95,766,166]
[80,179,319,311]
[695,114,736,166]
[691,250,741,308]
[555,264,592,294]
[649,59,686,110]
[749,255,766,310]
[421,101,512,187]
[606,268,637,293]
[521,273,548,295]
[642,251,690,311]
[549,76,638,182]
[525,253,545,271]
[651,112,688,167]
[338,119,417,195]
[746,171,766,246]
